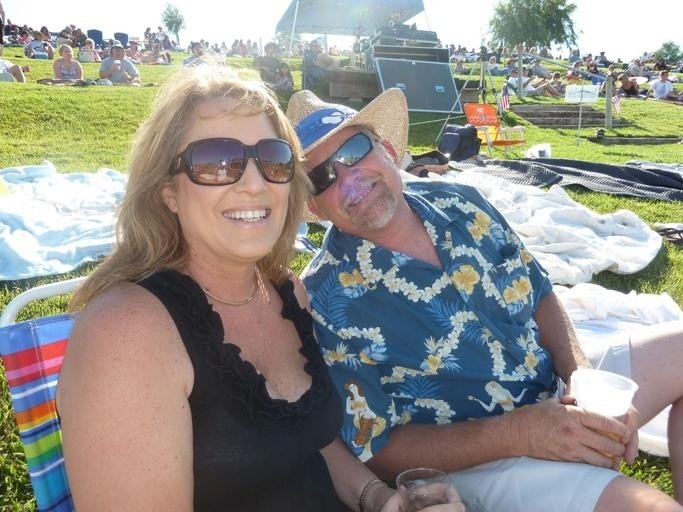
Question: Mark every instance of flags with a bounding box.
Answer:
[613,89,621,114]
[501,85,510,111]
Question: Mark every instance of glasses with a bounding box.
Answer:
[307,131,374,197]
[169,138,294,186]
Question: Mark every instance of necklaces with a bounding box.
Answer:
[201,270,271,306]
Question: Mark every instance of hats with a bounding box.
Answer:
[110,40,124,52]
[286,88,409,223]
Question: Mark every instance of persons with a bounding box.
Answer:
[438,40,683,102]
[54,59,465,511]
[389,10,406,28]
[0,7,187,87]
[286,85,682,510]
[187,38,360,103]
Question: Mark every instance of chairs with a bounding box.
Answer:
[463,103,526,159]
[0,275,87,512]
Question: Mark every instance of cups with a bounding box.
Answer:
[396,468,449,511]
[569,368,639,458]
[114,59,121,70]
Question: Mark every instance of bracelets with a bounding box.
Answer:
[359,478,383,510]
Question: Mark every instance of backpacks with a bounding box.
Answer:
[439,124,482,162]
